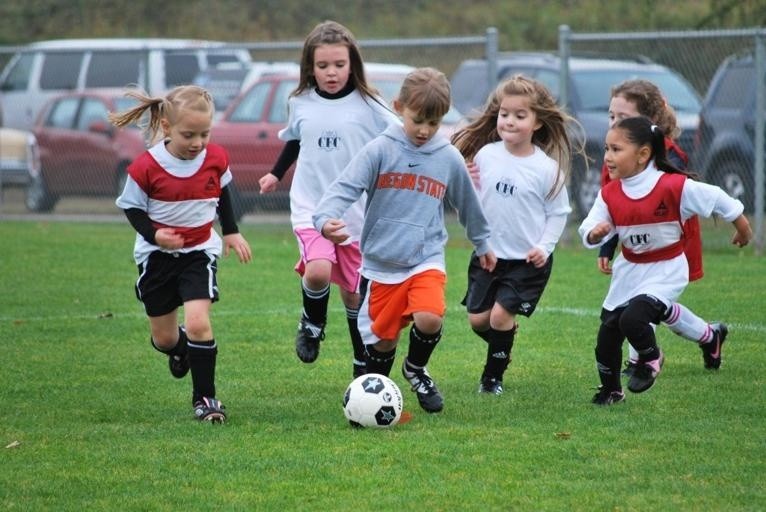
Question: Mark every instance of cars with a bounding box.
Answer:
[0,124,34,188]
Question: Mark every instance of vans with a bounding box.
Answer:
[0,38,252,134]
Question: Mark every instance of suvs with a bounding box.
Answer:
[449,47,706,219]
[693,53,766,216]
[204,62,473,225]
[23,88,160,211]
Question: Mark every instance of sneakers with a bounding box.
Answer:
[194,397,227,425]
[295,308,328,364]
[351,363,368,379]
[476,373,504,399]
[620,361,638,376]
[628,345,667,394]
[589,385,625,406]
[171,324,192,378]
[700,322,728,369]
[402,356,446,412]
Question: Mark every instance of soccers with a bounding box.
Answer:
[341,373,404,431]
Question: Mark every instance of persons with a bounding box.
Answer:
[257,20,403,382]
[578,116,753,407]
[105,86,251,423]
[597,80,705,377]
[446,75,598,396]
[311,65,500,412]
